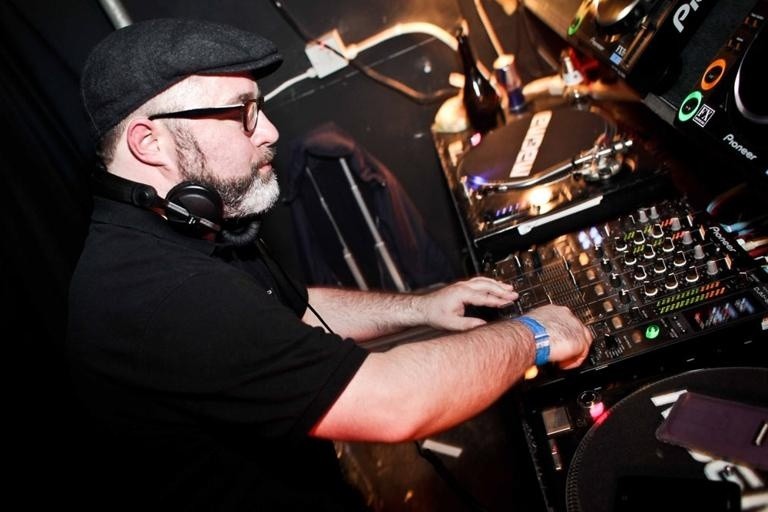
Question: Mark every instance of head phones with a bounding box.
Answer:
[95,170,226,242]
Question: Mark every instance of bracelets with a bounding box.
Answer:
[505,313,551,367]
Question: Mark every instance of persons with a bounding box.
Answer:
[59,17,596,512]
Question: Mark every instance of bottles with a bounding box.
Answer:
[562,57,592,110]
[456,35,506,134]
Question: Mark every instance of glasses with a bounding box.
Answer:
[146,93,267,136]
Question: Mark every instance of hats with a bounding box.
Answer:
[76,13,284,137]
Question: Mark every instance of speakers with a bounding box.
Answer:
[522,0,768,196]
